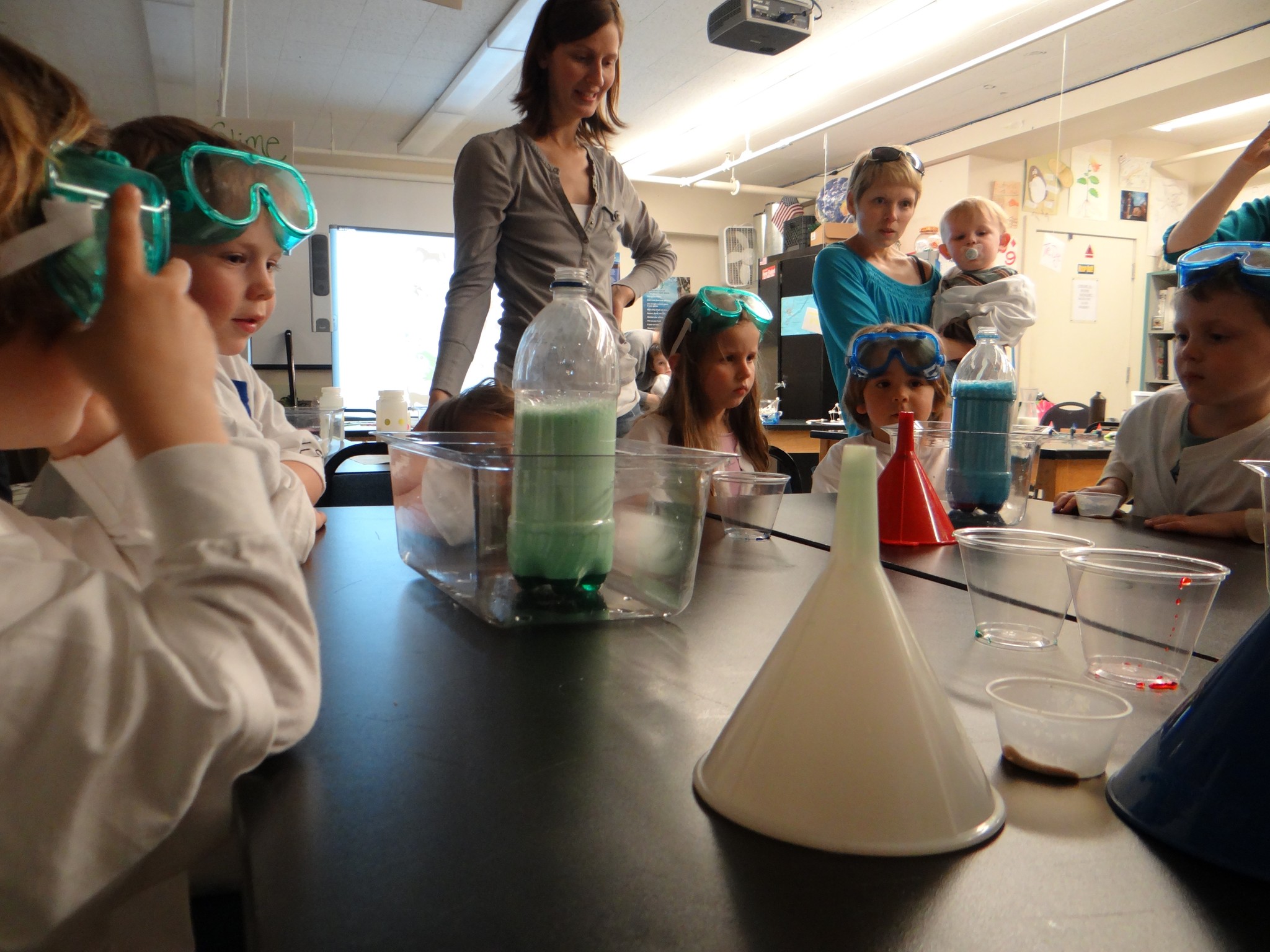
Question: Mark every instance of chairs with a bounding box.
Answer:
[1039,402,1090,432]
[313,442,394,507]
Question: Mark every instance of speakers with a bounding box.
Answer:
[308,232,334,333]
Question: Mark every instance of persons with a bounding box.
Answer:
[413,0,677,437]
[394,112,1270,540]
[1,35,326,952]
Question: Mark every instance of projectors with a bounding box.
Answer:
[707,0,814,56]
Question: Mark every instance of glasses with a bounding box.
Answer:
[686,286,773,340]
[847,331,944,379]
[862,147,925,180]
[1176,240,1270,288]
[150,141,318,255]
[40,142,172,319]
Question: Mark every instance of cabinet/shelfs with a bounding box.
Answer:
[1140,270,1180,391]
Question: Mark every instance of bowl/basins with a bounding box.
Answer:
[879,419,1055,527]
[368,430,743,631]
[285,408,345,458]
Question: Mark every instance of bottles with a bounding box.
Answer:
[506,266,621,598]
[946,326,1017,514]
[319,387,343,439]
[1089,392,1106,426]
[376,389,411,431]
[914,227,943,252]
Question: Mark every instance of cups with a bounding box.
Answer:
[712,471,792,540]
[1074,491,1123,519]
[758,399,780,425]
[1017,401,1038,426]
[986,676,1134,780]
[1059,546,1231,690]
[954,526,1095,650]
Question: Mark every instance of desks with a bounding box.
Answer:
[239,494,1270,952]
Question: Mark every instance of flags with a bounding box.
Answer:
[771,195,804,233]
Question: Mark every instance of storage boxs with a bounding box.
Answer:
[783,215,819,253]
[810,222,858,247]
